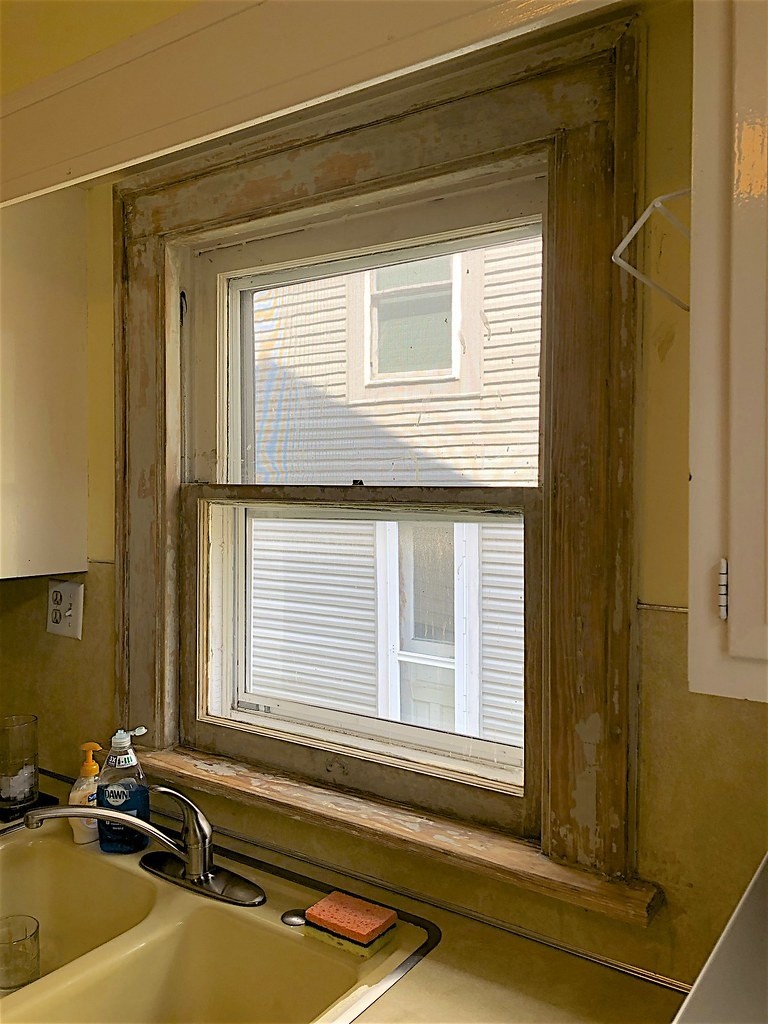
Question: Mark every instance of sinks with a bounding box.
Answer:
[1,816,189,1023]
[4,894,441,1024]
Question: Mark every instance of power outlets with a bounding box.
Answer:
[44,578,87,641]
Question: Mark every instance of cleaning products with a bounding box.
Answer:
[99,725,148,857]
[67,742,102,844]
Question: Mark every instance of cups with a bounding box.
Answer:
[0,714,40,813]
[0,915,40,999]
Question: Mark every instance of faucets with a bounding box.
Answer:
[22,782,270,909]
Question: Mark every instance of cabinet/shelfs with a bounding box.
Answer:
[683,0,768,707]
